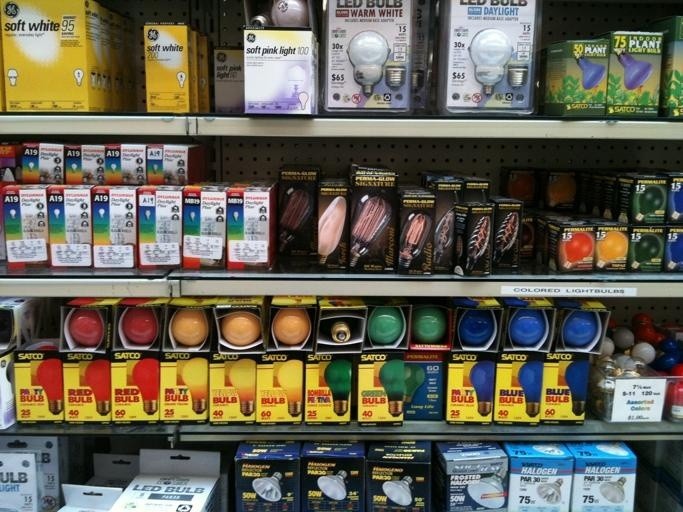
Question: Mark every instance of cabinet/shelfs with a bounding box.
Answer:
[0,109,682,443]
